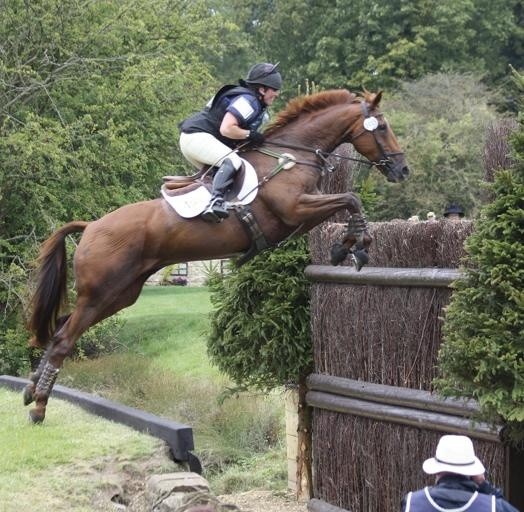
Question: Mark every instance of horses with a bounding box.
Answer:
[23,87,412,426]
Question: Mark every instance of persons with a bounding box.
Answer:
[400,433,519,512]
[179,63,281,220]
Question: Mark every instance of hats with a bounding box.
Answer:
[422,434,486,477]
[443,205,464,218]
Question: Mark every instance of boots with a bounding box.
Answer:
[201,158,237,221]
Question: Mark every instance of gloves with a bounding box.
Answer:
[245,129,265,146]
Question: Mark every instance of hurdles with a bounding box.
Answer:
[305,220,519,512]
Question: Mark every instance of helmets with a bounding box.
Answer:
[245,61,282,90]
